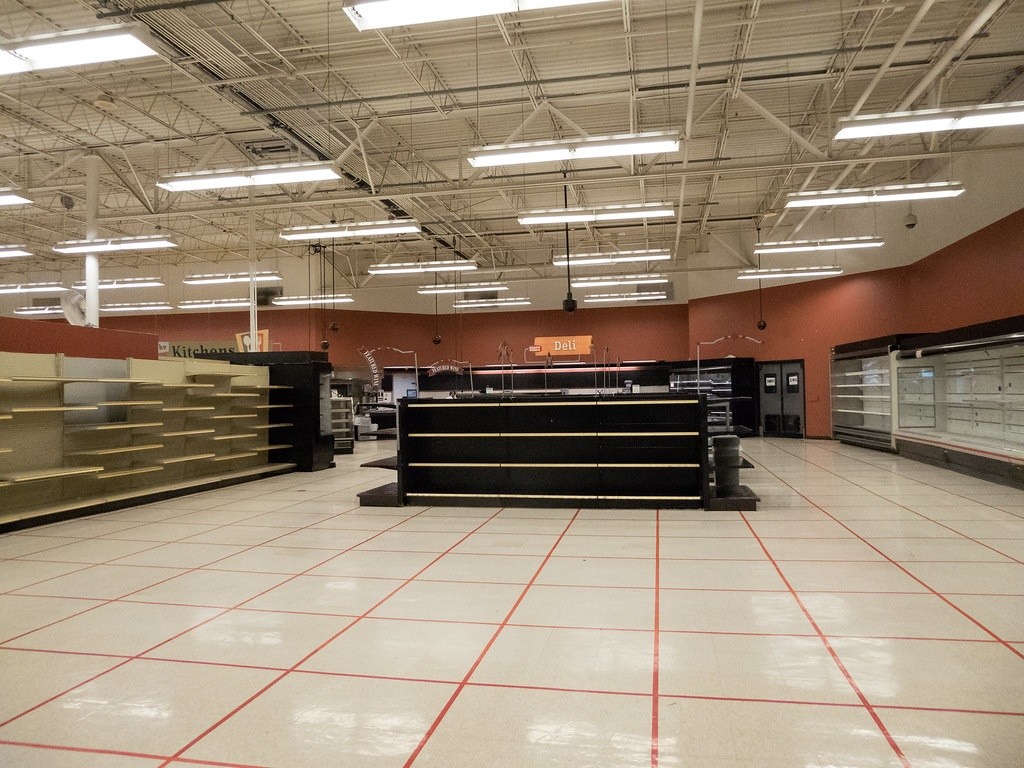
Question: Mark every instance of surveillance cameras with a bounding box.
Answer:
[321,341,329,350]
[563,300,577,314]
[432,335,441,344]
[904,214,917,229]
[329,323,339,332]
[757,321,766,330]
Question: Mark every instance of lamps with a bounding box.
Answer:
[342,0,611,33]
[0,102,680,315]
[738,80,1024,279]
[0,17,160,76]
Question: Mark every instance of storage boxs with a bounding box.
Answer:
[358,424,378,440]
[354,417,371,425]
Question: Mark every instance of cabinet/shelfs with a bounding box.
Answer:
[829,314,1024,490]
[356,357,761,511]
[0,351,356,534]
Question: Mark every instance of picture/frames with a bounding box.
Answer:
[407,389,417,398]
